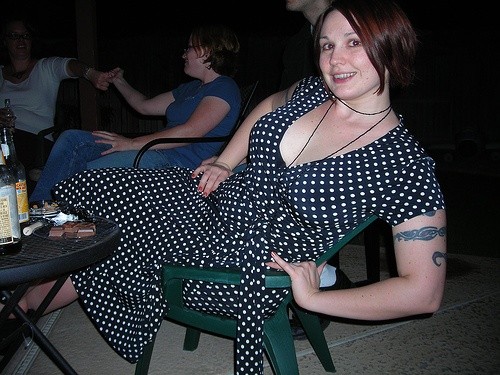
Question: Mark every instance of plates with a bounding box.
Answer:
[27,200,61,218]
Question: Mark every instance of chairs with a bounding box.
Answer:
[35,81,374,375]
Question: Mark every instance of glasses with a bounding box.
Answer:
[2,31,34,40]
[184,45,199,53]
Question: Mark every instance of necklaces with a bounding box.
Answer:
[270,95,392,199]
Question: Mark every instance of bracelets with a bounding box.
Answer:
[84,66,92,78]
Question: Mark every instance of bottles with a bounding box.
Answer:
[4,98,16,133]
[0,140,23,257]
[0,127,30,227]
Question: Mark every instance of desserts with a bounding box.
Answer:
[48,221,97,237]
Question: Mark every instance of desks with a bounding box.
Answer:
[0,217,120,375]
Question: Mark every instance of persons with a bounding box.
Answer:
[0,16,116,164]
[0,0,447,323]
[25,25,243,207]
[281,0,334,90]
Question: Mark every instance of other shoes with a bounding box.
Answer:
[284,268,355,340]
